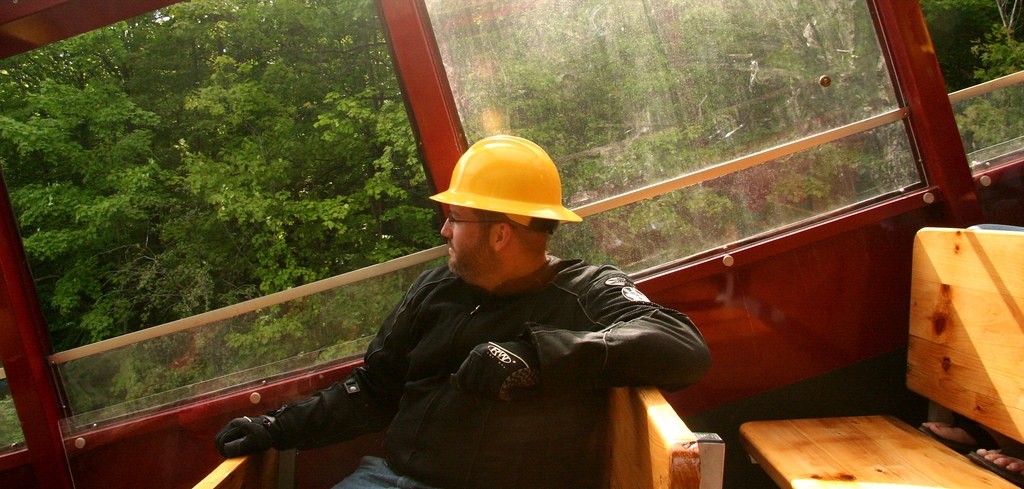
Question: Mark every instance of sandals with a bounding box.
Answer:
[969,449,1024,489]
[918,421,998,455]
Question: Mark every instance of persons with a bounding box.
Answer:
[215,134,713,489]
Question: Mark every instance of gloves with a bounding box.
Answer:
[455,341,540,404]
[215,415,280,459]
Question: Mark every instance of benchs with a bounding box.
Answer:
[594,385,725,489]
[191,448,301,489]
[738,228,1024,489]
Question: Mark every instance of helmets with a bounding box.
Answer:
[429,135,583,222]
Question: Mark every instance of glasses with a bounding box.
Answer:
[447,213,514,228]
